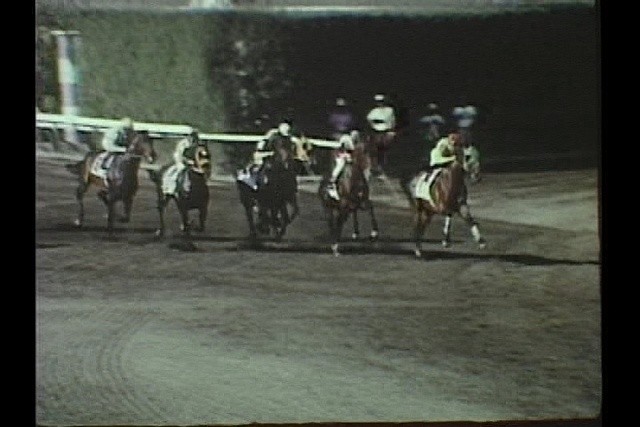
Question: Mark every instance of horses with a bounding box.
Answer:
[399,138,488,258]
[232,131,301,245]
[63,129,158,240]
[318,136,379,251]
[145,137,213,251]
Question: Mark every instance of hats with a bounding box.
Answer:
[337,98,345,105]
[278,123,290,136]
[375,94,384,100]
[121,117,131,127]
[430,103,437,108]
[351,130,359,135]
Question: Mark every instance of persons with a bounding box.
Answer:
[420,101,444,132]
[174,131,200,198]
[327,130,360,203]
[100,118,134,185]
[253,123,293,186]
[367,93,397,179]
[455,95,479,131]
[330,97,353,130]
[430,133,466,170]
